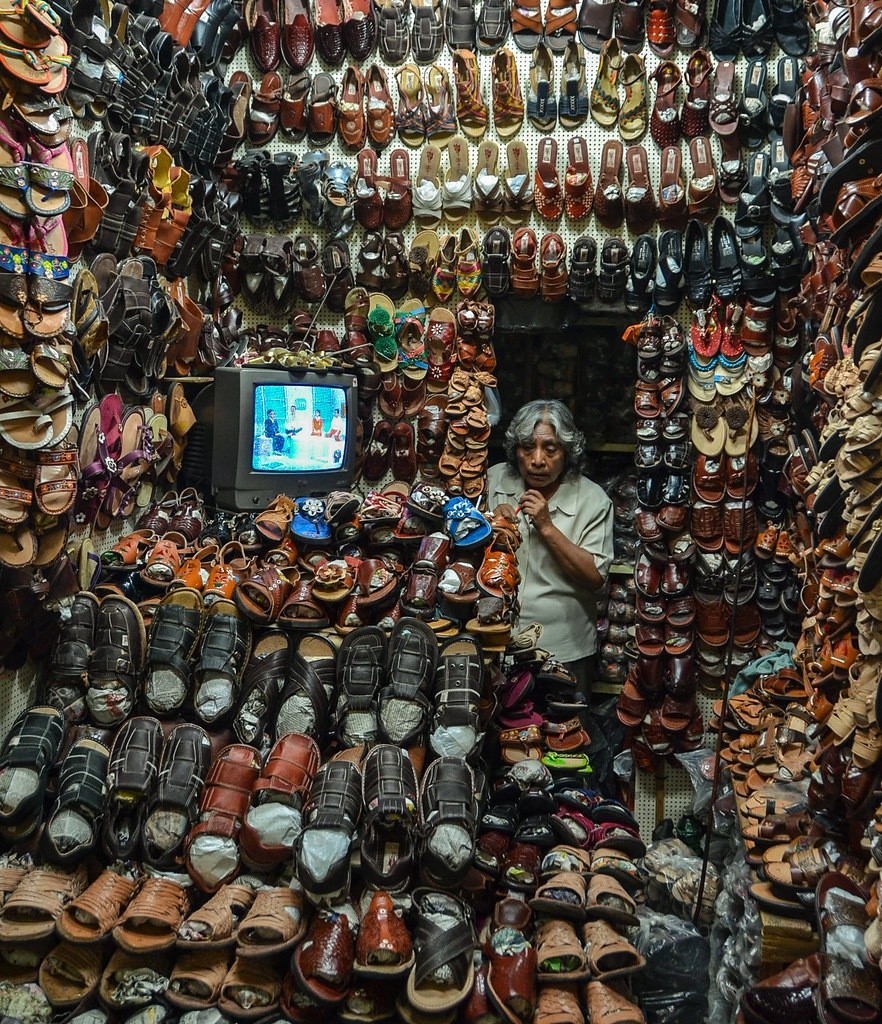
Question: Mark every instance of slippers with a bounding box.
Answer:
[411,136,771,238]
[710,60,766,135]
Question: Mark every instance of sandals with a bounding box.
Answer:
[591,38,625,127]
[676,0,710,48]
[337,66,367,147]
[709,0,769,62]
[544,0,580,52]
[395,67,426,148]
[646,61,682,149]
[236,149,411,232]
[281,68,312,141]
[248,71,283,147]
[423,65,457,150]
[445,0,476,57]
[379,617,439,747]
[335,626,388,746]
[245,482,520,630]
[578,0,617,55]
[510,0,543,53]
[608,303,882,1023]
[482,624,647,1022]
[2,706,478,880]
[769,0,882,350]
[1,865,482,1024]
[374,0,410,64]
[230,630,293,745]
[559,44,590,129]
[410,0,444,66]
[647,0,676,57]
[477,0,511,55]
[615,0,646,54]
[683,49,715,141]
[146,587,205,719]
[492,54,524,138]
[456,48,490,138]
[238,217,770,312]
[343,287,497,497]
[272,633,337,748]
[430,636,479,764]
[619,53,650,140]
[88,593,147,722]
[193,599,252,726]
[528,43,557,132]
[308,73,339,146]
[365,65,395,147]
[46,591,100,721]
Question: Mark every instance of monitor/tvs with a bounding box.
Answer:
[165,275,371,511]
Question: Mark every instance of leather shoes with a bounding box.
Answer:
[343,0,377,61]
[279,0,315,73]
[313,0,344,66]
[245,0,282,74]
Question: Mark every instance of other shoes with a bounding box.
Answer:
[0,0,254,603]
[245,312,340,373]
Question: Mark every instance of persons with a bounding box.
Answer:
[488,400,614,728]
[266,405,343,458]
